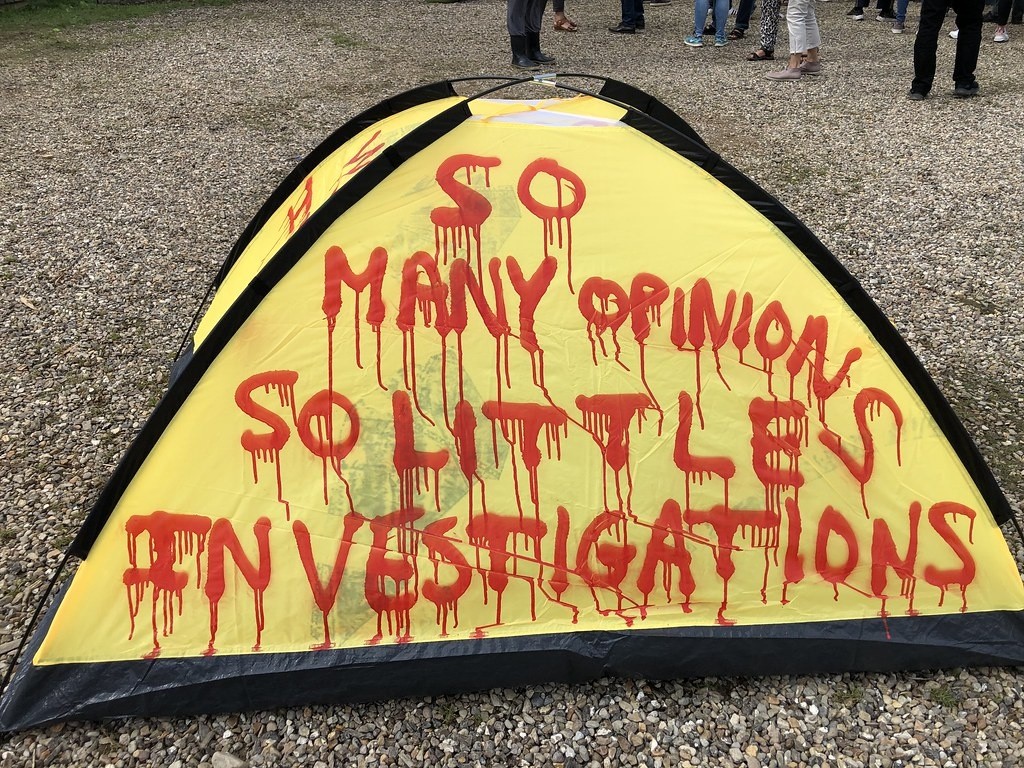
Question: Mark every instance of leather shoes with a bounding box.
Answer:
[609,21,644,35]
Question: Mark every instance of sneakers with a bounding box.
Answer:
[994,27,1009,42]
[847,7,864,21]
[728,7,738,15]
[714,34,728,47]
[876,9,898,22]
[684,34,703,47]
[948,29,959,38]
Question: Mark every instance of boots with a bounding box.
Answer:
[511,32,555,70]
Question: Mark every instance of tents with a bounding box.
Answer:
[0,71,1024,742]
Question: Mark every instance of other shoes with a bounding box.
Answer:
[891,19,903,33]
[908,87,923,100]
[766,61,820,82]
[693,24,716,35]
[983,12,1007,25]
[727,28,743,39]
[649,0,672,6]
[954,82,979,98]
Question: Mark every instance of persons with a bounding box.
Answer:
[693,0,755,40]
[608,0,645,34]
[765,0,821,81]
[847,0,919,36]
[982,0,1024,24]
[906,0,980,101]
[948,0,1010,42]
[684,0,729,47]
[506,0,557,70]
[745,0,819,60]
[552,0,578,32]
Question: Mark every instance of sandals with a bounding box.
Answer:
[554,16,579,32]
[745,48,775,60]
